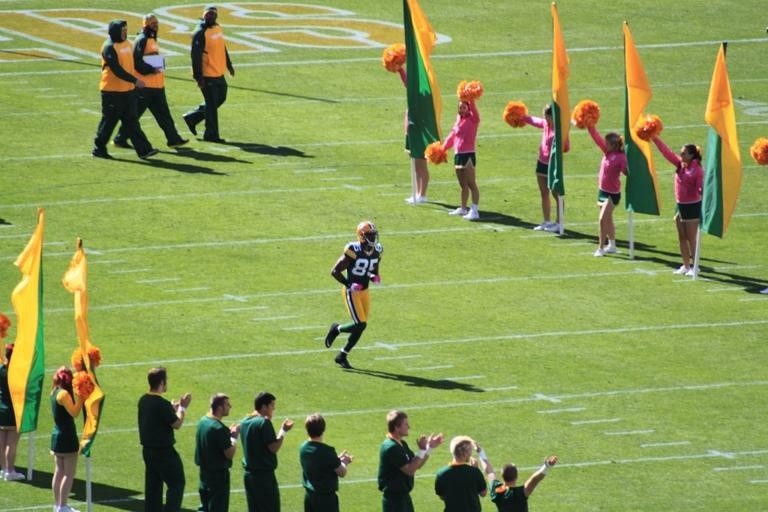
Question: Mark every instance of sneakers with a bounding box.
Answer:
[533,223,551,231]
[409,195,427,204]
[204,136,226,144]
[114,138,133,149]
[1,470,23,478]
[463,209,479,220]
[605,246,616,253]
[325,324,338,347]
[686,269,699,276]
[593,250,606,256]
[760,287,768,294]
[167,139,189,148]
[4,470,25,481]
[674,266,691,275]
[52,505,58,512]
[58,506,81,512]
[405,194,416,202]
[92,148,113,159]
[139,149,158,159]
[334,355,353,368]
[182,113,197,135]
[448,207,467,215]
[544,225,562,232]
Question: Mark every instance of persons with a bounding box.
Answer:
[139,366,191,512]
[436,436,486,512]
[91,20,160,159]
[423,81,483,220]
[183,7,237,145]
[397,65,429,203]
[473,440,557,512]
[240,393,294,512]
[325,220,384,370]
[195,395,240,512]
[571,97,633,258]
[300,414,352,512]
[49,366,94,512]
[377,409,443,512]
[114,15,188,147]
[0,344,26,482]
[503,102,569,233]
[636,114,709,278]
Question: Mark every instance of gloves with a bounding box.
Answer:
[351,283,362,292]
[372,275,381,285]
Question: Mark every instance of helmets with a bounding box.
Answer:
[357,222,378,244]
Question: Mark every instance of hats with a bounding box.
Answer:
[142,15,159,27]
[202,5,219,19]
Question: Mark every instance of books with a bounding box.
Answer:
[142,51,166,72]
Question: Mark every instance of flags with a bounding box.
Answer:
[403,0,442,158]
[700,42,742,237]
[546,1,569,195]
[8,207,44,437]
[623,21,660,217]
[63,238,105,461]
